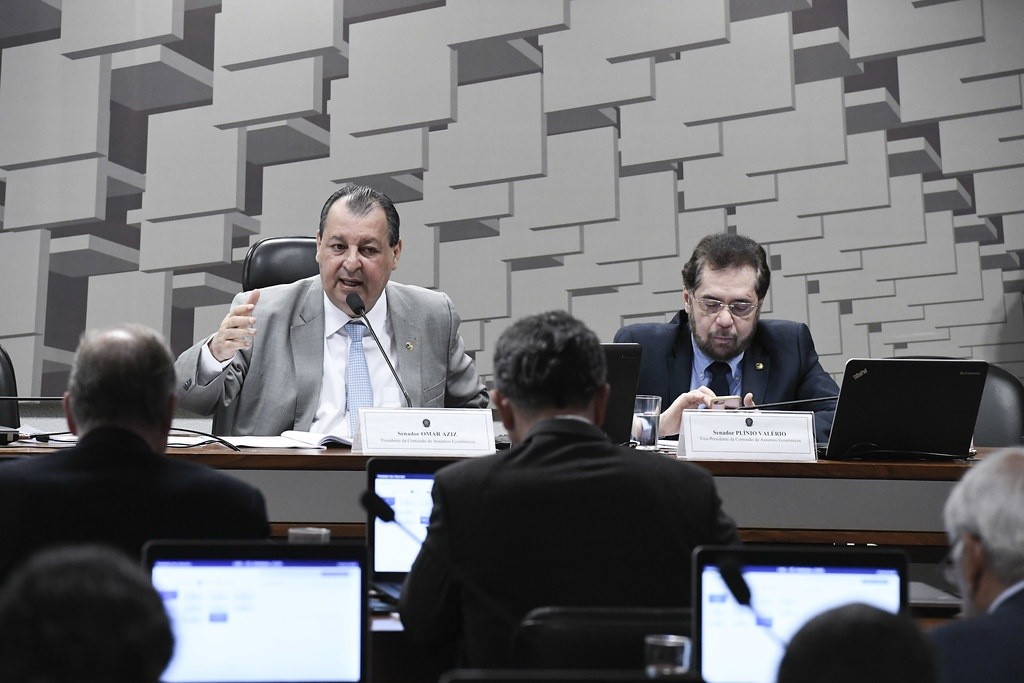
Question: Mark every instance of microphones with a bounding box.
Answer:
[361,492,422,544]
[346,293,412,407]
[715,560,787,651]
[35,434,78,443]
[725,396,839,410]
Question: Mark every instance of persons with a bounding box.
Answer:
[0,323,274,573]
[175,183,491,436]
[612,233,840,441]
[775,604,941,683]
[399,308,745,667]
[928,446,1024,683]
[0,542,177,683]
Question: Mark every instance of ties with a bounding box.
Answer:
[344,320,375,439]
[706,361,732,395]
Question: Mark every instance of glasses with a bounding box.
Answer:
[689,291,765,317]
[940,535,973,586]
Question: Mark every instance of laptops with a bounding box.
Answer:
[494,343,645,452]
[142,540,370,683]
[691,544,909,683]
[814,359,989,460]
[366,455,463,605]
[907,583,963,616]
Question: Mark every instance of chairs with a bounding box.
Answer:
[241,236,320,291]
[878,353,1023,618]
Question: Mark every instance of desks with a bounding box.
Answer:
[0,431,984,559]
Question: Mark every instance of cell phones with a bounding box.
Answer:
[710,396,741,411]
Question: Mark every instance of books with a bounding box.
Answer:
[219,430,353,449]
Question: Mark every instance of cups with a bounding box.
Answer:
[644,633,691,676]
[287,526,331,545]
[635,394,661,451]
